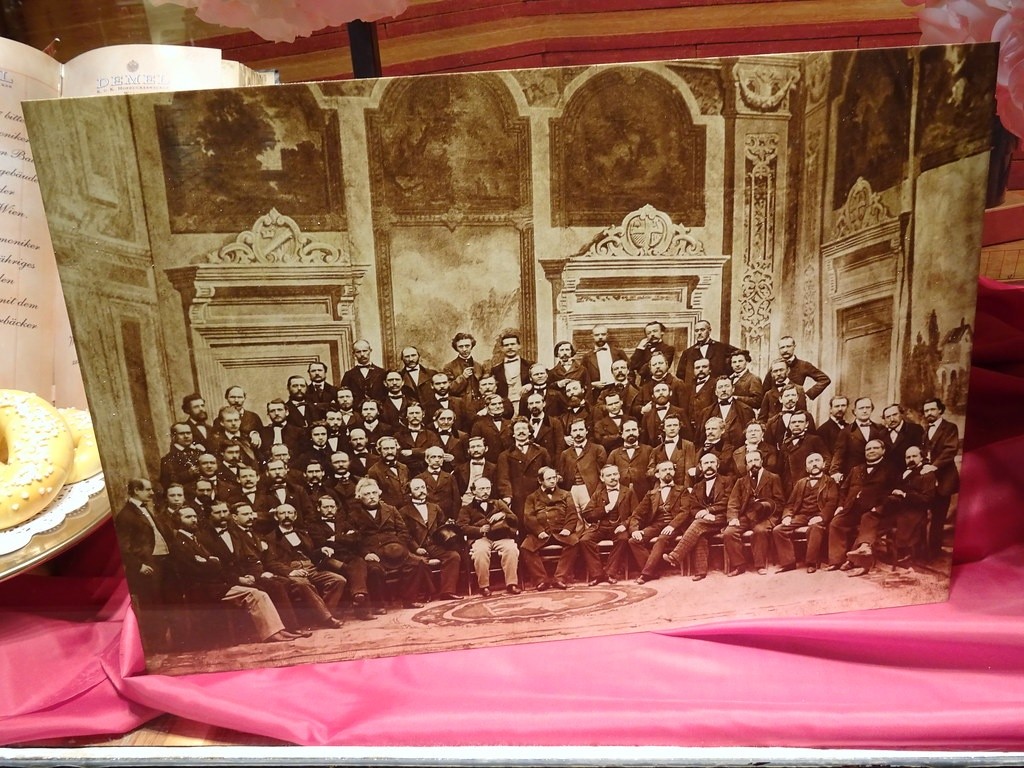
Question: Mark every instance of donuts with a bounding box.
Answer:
[0,389,73,530]
[56,408,103,485]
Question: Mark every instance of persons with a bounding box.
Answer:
[117,319,961,645]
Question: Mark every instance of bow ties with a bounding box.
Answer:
[859,422,872,427]
[140,343,936,522]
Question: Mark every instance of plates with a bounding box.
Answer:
[0,472,112,582]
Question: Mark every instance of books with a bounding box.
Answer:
[1,37,281,408]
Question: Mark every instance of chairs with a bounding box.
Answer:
[168,511,933,644]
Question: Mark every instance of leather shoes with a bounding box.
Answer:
[807,563,816,573]
[663,553,678,567]
[727,568,744,576]
[774,564,796,574]
[552,581,567,589]
[692,575,705,581]
[637,571,659,584]
[823,560,854,572]
[846,543,873,558]
[848,566,868,576]
[360,612,378,620]
[403,602,425,608]
[440,593,464,600]
[320,616,343,628]
[292,629,312,638]
[264,630,301,642]
[604,575,617,584]
[482,587,491,597]
[507,585,521,594]
[758,567,767,574]
[373,606,387,615]
[349,593,365,609]
[588,576,603,586]
[537,580,550,590]
